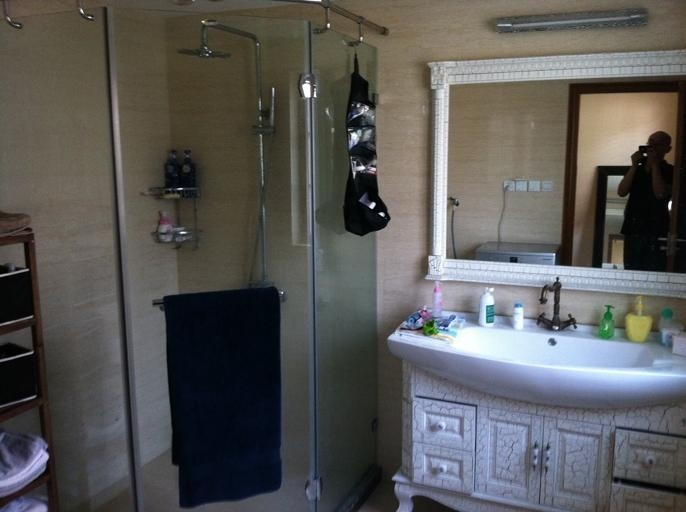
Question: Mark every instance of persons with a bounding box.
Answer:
[617,131,674,272]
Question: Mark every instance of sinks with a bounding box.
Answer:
[461,327,680,370]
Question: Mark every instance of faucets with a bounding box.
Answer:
[540,276,561,330]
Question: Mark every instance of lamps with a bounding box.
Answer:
[494,7,649,34]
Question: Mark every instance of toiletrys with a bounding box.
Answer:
[432,281,442,318]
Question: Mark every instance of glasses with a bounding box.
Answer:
[647,141,665,147]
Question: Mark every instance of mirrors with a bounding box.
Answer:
[425,48,685,299]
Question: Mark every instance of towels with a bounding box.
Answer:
[161,285,282,509]
[0,427,50,512]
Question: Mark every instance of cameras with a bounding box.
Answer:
[639,146,650,153]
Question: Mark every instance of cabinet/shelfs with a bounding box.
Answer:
[144,186,203,246]
[391,357,686,512]
[0,227,62,512]
